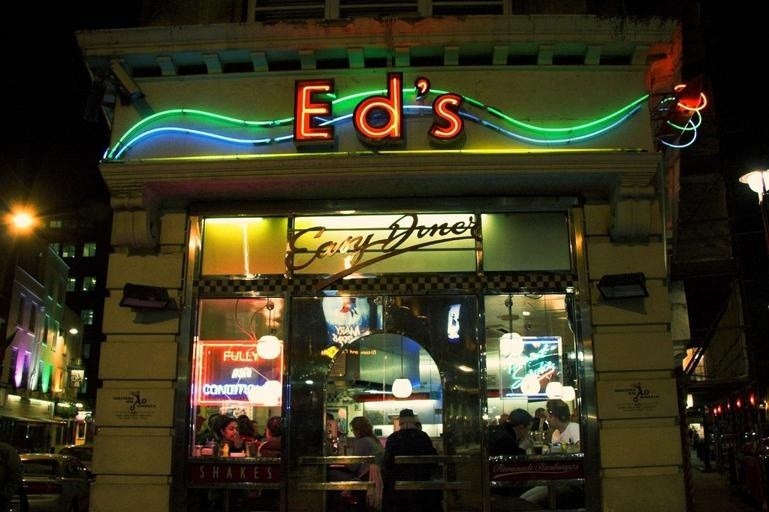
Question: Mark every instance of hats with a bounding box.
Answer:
[394,408,418,418]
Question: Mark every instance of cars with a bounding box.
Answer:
[716,432,769,468]
[730,429,769,512]
[58,443,92,473]
[8,447,89,512]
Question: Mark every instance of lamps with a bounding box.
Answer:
[119,283,178,312]
[597,272,649,301]
[256,301,281,360]
[392,335,413,399]
[500,297,576,403]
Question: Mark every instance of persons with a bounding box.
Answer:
[331,409,439,507]
[486,400,583,508]
[0,442,30,512]
[199,414,282,508]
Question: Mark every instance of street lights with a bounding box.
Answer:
[0,173,37,367]
[735,161,769,245]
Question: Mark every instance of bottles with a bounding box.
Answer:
[213,442,229,457]
[549,438,578,452]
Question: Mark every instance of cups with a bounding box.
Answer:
[246,441,258,457]
[192,444,212,456]
[533,440,543,455]
